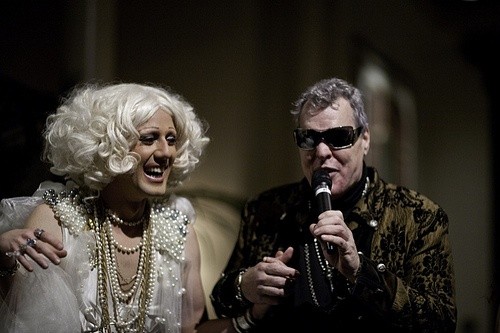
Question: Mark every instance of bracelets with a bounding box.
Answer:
[232,309,256,333]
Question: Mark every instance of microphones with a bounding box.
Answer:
[311,169,335,255]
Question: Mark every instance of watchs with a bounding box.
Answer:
[236,271,245,298]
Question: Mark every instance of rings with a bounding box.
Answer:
[36,228,44,237]
[27,239,38,246]
[22,244,28,251]
[16,252,21,257]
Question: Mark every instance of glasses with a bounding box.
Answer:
[293,126,363,150]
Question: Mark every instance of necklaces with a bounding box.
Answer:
[91,202,155,333]
[304,238,347,307]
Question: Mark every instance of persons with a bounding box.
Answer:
[209,78,459,333]
[0,83,293,333]
